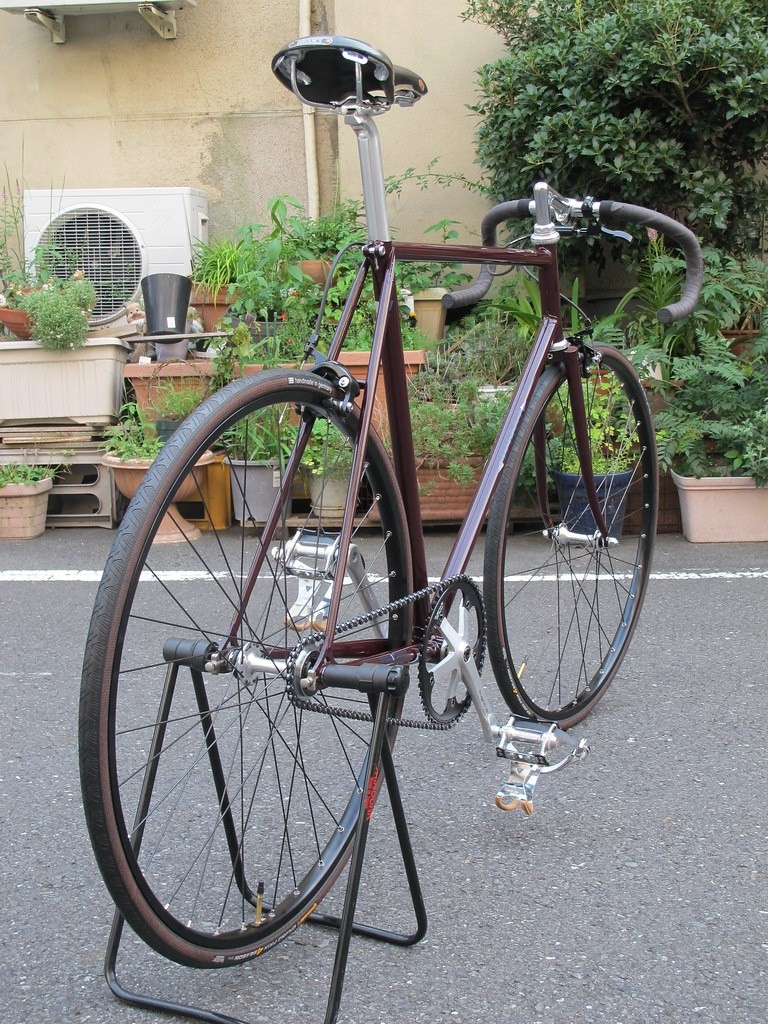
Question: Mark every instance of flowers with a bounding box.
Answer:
[227,271,302,345]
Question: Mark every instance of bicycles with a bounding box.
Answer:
[74,33,706,1012]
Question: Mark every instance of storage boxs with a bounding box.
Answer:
[178,453,231,530]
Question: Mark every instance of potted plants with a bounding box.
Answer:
[0,178,768,543]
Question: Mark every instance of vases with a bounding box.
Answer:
[226,308,287,351]
[22,285,43,296]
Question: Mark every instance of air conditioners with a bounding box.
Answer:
[22,185,209,330]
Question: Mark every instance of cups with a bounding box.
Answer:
[140,274,193,345]
